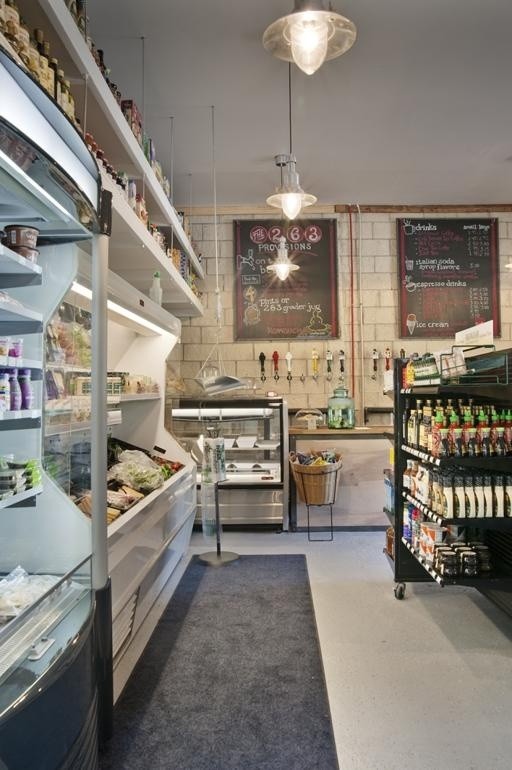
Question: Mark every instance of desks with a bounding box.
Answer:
[290,422,395,531]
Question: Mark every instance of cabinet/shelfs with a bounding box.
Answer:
[382,389,395,571]
[1,56,100,770]
[45,245,197,721]
[1,1,205,310]
[171,398,285,532]
[401,368,512,586]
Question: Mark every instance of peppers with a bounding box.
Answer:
[161,462,181,479]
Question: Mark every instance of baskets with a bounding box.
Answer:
[288,450,344,505]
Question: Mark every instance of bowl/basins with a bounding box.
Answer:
[207,439,234,448]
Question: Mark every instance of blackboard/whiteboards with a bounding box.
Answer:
[233,219,338,340]
[395,218,501,339]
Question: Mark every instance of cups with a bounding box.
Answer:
[9,339,24,358]
[0,336,11,355]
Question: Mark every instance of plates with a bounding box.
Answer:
[255,439,280,447]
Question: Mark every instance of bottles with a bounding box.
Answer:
[0,0,75,121]
[149,271,162,305]
[402,397,512,458]
[410,461,418,496]
[328,389,355,428]
[403,459,413,493]
[17,369,32,409]
[0,373,10,410]
[0,368,22,410]
[85,132,137,211]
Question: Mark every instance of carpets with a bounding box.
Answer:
[98,554,342,770]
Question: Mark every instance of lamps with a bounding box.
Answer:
[262,1,358,75]
[265,154,301,281]
[265,62,316,223]
